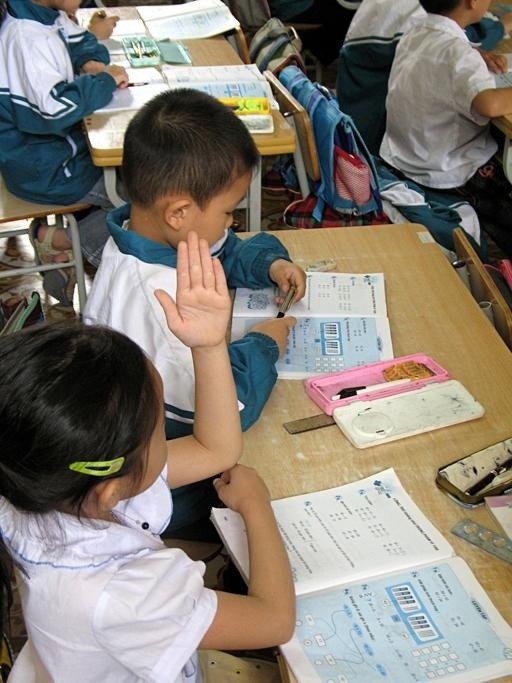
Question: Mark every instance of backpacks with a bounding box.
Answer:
[250,17,306,77]
[274,66,388,229]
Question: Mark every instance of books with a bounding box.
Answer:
[230,271,393,380]
[489,52,512,89]
[72,0,280,113]
[211,465,512,683]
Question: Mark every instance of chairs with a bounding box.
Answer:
[1,175,92,315]
[233,0,512,344]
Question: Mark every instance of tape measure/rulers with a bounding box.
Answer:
[451,517,512,565]
[283,413,335,434]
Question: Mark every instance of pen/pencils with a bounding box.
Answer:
[331,378,411,399]
[131,40,155,57]
[465,457,512,496]
[127,82,149,87]
[277,287,296,318]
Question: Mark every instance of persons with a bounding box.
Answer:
[84,9,119,41]
[1,229,296,683]
[335,0,512,156]
[377,0,512,259]
[0,0,135,307]
[76,88,307,541]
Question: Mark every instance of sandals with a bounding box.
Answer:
[27,216,78,306]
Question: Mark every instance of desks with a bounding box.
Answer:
[224,221,511,682]
[61,4,296,232]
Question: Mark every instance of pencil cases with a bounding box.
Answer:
[304,353,485,449]
[122,36,191,67]
[215,97,274,134]
[435,437,512,509]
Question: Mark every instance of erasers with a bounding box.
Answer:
[305,258,337,272]
